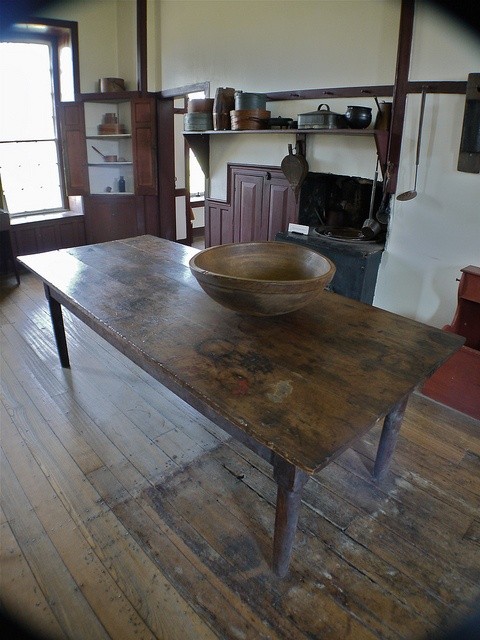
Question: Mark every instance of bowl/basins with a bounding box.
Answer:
[189,241,337,317]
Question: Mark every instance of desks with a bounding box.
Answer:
[16,235,466,578]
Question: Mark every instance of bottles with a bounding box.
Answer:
[119,175,126,192]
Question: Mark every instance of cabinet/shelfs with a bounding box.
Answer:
[54,92,158,245]
[203,199,229,251]
[228,165,300,243]
